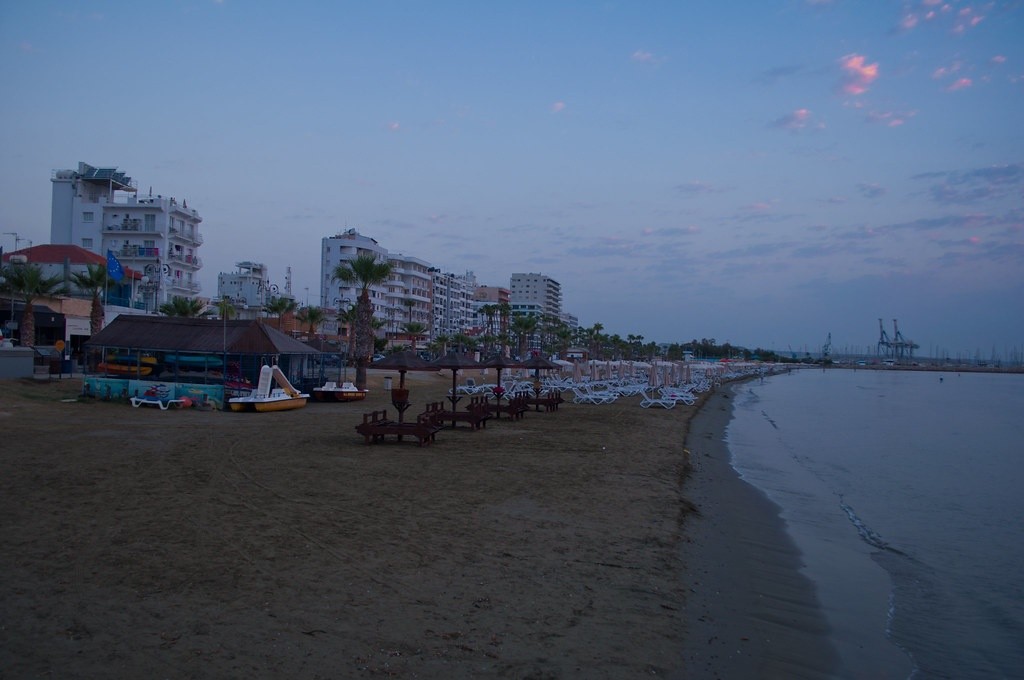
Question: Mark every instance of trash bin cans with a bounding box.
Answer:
[2,339,14,349]
[384,377,392,392]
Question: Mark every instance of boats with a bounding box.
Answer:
[312,381,368,402]
[228,365,310,413]
[98,350,226,385]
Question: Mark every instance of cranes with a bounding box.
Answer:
[876,318,920,360]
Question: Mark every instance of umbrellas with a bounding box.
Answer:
[550,359,769,399]
[368,350,556,441]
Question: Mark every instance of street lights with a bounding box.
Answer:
[255,276,281,326]
[141,256,171,315]
[333,293,353,354]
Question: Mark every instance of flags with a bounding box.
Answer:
[107,250,124,284]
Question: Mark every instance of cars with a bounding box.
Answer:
[856,361,867,367]
[372,353,386,362]
[419,353,430,361]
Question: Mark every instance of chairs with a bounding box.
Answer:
[449,369,746,409]
[130,396,185,410]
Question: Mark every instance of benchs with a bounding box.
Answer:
[355,387,564,447]
